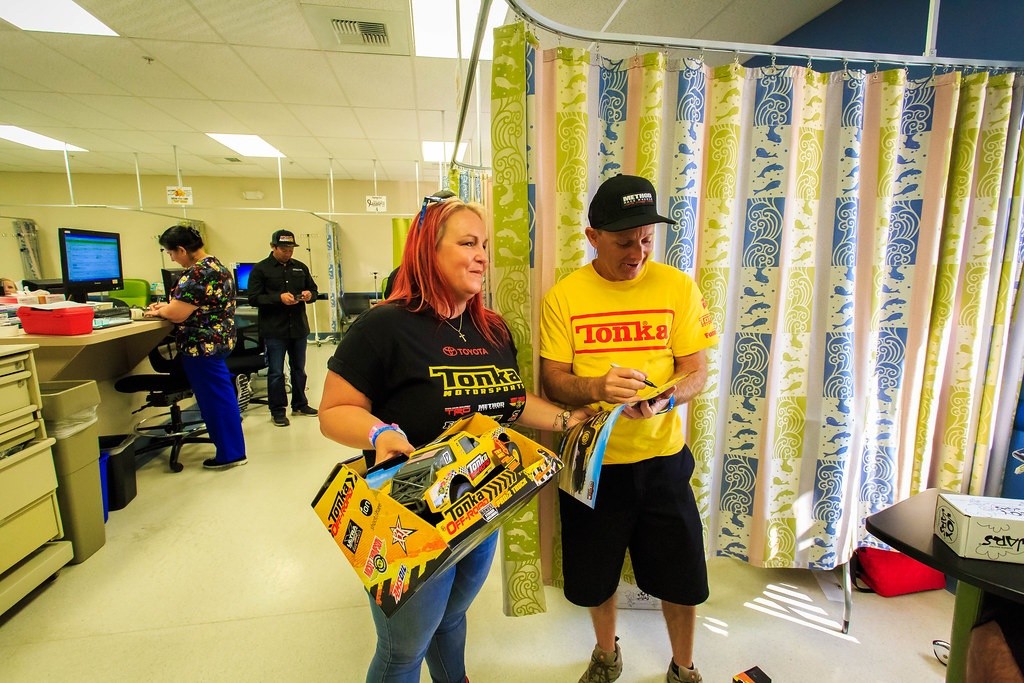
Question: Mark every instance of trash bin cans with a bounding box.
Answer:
[97,453,111,524]
[98,434,139,513]
[37,380,106,566]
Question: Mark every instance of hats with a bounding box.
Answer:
[589,174,676,232]
[272,229,299,247]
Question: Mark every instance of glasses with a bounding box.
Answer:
[418,190,457,231]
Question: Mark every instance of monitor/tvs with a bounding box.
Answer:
[234,262,257,289]
[161,267,187,300]
[58,227,124,305]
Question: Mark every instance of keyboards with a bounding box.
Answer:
[94,308,131,319]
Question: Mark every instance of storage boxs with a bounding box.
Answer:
[17,307,95,335]
[934,493,1024,564]
[310,411,565,620]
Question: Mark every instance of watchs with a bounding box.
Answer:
[557,408,571,431]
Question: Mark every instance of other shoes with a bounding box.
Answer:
[203,456,248,469]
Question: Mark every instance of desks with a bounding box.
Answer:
[866,487,1024,683]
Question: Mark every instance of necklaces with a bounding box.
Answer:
[421,297,467,343]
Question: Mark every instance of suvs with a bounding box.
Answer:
[385,428,525,523]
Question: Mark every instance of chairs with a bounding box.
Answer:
[841,542,947,634]
[116,328,270,472]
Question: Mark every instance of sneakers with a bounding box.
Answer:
[271,415,290,426]
[667,656,703,683]
[578,637,623,683]
[291,404,318,416]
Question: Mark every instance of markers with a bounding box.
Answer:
[610,364,657,388]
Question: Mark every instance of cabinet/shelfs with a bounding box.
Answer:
[0,344,64,576]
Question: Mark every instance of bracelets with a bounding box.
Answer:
[368,421,408,447]
[656,395,675,414]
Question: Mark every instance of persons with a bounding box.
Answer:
[0,278,18,295]
[248,229,318,427]
[317,191,605,683]
[143,226,250,470]
[542,173,718,683]
